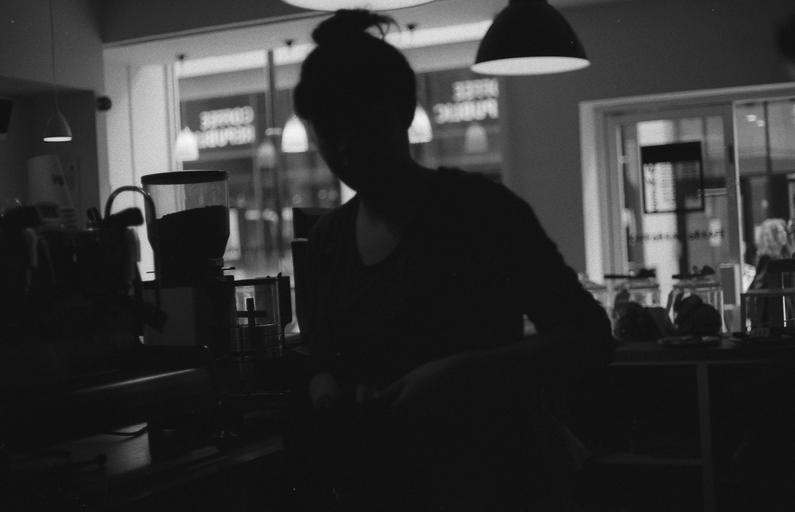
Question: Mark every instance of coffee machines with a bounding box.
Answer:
[138,164,299,431]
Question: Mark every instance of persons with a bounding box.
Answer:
[293,7,617,510]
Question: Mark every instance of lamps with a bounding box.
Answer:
[43,1,72,143]
[469,1,591,75]
